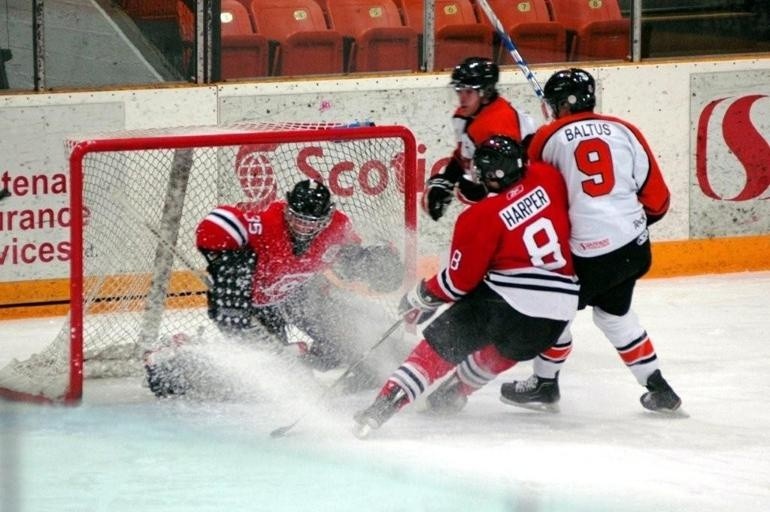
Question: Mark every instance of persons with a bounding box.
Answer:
[147,179,402,401]
[350,135,581,439]
[418,56,539,222]
[500,67,681,414]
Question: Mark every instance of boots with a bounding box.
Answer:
[427,370,480,415]
[349,379,412,428]
[500,371,562,403]
[640,370,681,413]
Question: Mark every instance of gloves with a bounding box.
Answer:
[423,174,455,221]
[398,278,441,325]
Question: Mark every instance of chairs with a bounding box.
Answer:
[125,1,650,94]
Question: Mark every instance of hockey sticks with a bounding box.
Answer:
[272,318,405,441]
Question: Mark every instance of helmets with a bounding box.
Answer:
[450,56,499,92]
[473,135,528,191]
[542,68,596,119]
[285,179,336,255]
